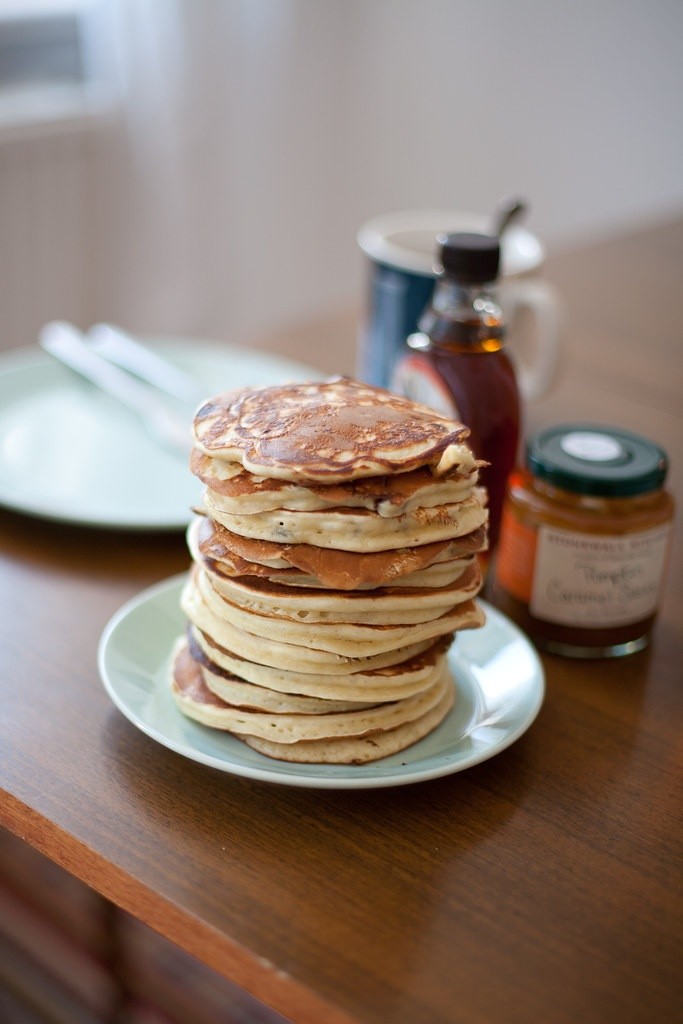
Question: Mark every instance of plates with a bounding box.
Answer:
[0,343,334,532]
[99,574,546,790]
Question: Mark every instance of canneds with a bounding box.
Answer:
[494,423,676,658]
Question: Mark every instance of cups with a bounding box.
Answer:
[359,213,560,403]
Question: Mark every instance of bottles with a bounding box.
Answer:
[387,232,523,595]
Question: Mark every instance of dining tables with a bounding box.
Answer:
[0,209,683,1024]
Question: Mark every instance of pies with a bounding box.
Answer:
[171,375,489,763]
